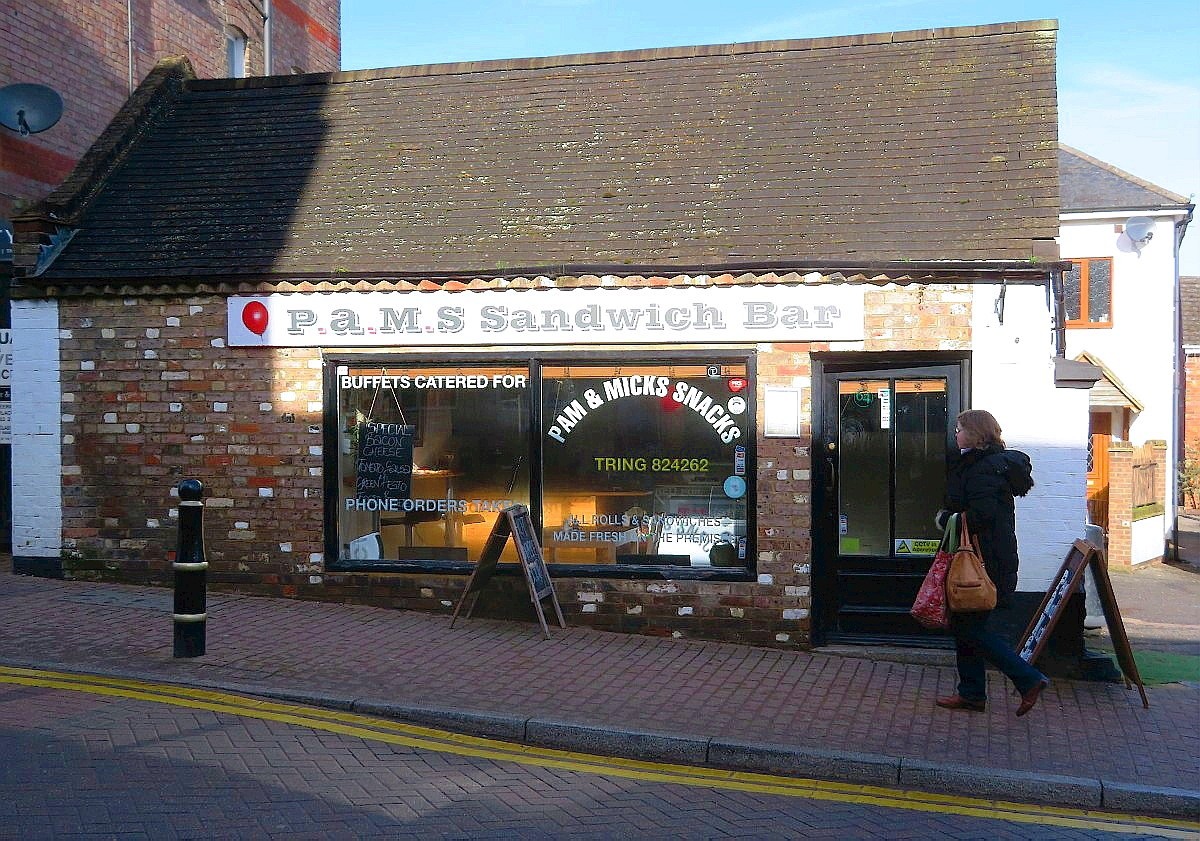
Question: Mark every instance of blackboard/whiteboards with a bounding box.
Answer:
[1019,539,1143,682]
[357,422,415,511]
[464,505,551,600]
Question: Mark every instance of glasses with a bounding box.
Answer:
[955,427,963,432]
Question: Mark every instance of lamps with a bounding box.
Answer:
[0,78,68,141]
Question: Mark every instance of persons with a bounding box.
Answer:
[936,411,1048,716]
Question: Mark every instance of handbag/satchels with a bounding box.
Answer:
[911,513,959,631]
[945,512,996,612]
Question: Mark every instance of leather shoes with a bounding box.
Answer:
[1016,674,1048,716]
[936,693,986,712]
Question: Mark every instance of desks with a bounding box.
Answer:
[542,524,639,565]
[365,469,465,547]
